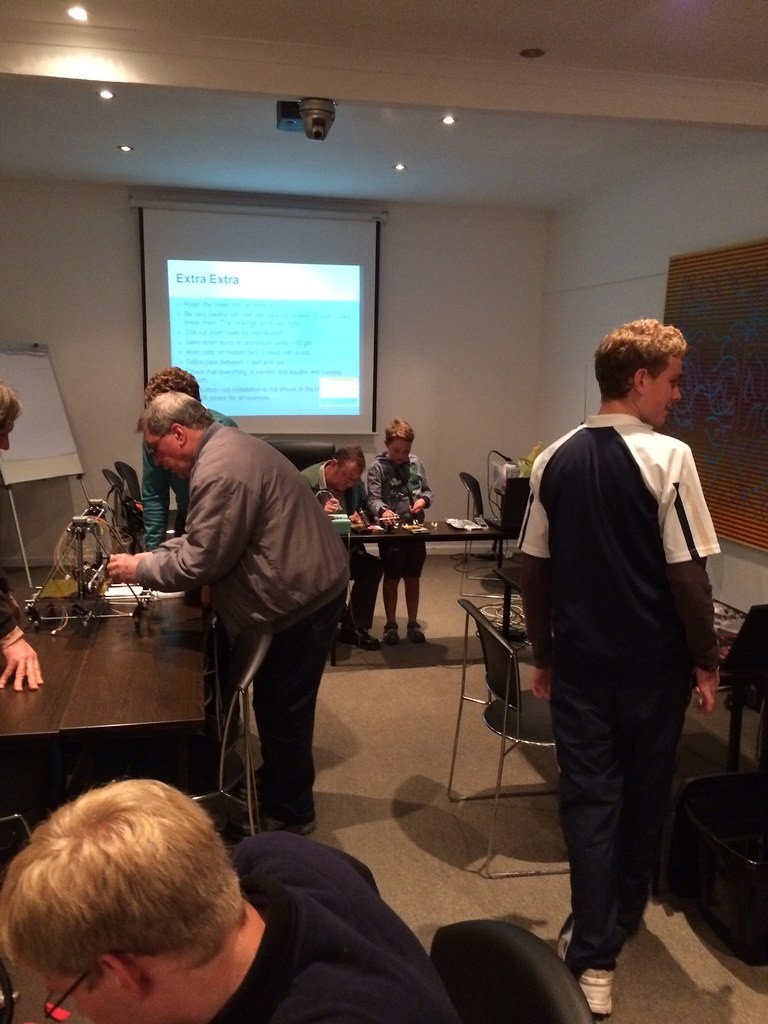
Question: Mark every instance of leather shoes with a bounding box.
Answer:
[340,623,380,650]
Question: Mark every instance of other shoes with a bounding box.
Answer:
[232,781,264,807]
[224,808,315,843]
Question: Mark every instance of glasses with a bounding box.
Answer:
[145,420,184,457]
[44,950,127,1022]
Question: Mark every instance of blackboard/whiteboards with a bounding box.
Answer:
[0,342,85,486]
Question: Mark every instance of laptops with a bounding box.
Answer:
[485,477,533,532]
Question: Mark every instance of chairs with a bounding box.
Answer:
[447,597,570,880]
[0,615,274,865]
[460,466,531,568]
[267,439,336,471]
[428,918,598,1024]
[666,690,768,967]
[103,461,181,555]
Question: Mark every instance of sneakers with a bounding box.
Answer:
[407,622,425,642]
[559,932,611,1017]
[382,623,399,645]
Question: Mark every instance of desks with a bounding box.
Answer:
[331,518,503,666]
[493,566,768,774]
[0,563,207,800]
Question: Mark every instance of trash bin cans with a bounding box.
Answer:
[667,770,768,966]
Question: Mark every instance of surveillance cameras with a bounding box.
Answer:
[301,99,337,141]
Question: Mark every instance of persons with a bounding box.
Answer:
[517,320,722,1014]
[0,381,43,690]
[367,420,433,643]
[300,446,380,651]
[1,780,464,1024]
[108,390,351,838]
[143,368,238,606]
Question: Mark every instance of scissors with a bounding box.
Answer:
[695,686,731,706]
